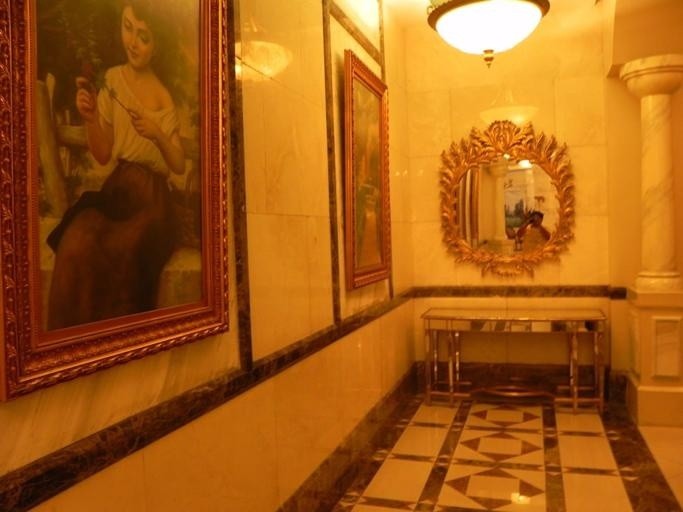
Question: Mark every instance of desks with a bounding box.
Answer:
[420,308,607,416]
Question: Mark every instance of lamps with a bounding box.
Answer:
[427,0,551,70]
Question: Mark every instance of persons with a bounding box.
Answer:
[517,212,550,254]
[46,0,186,332]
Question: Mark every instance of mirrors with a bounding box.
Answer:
[440,121,576,280]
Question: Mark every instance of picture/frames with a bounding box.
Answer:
[343,48,393,290]
[1,0,230,401]
